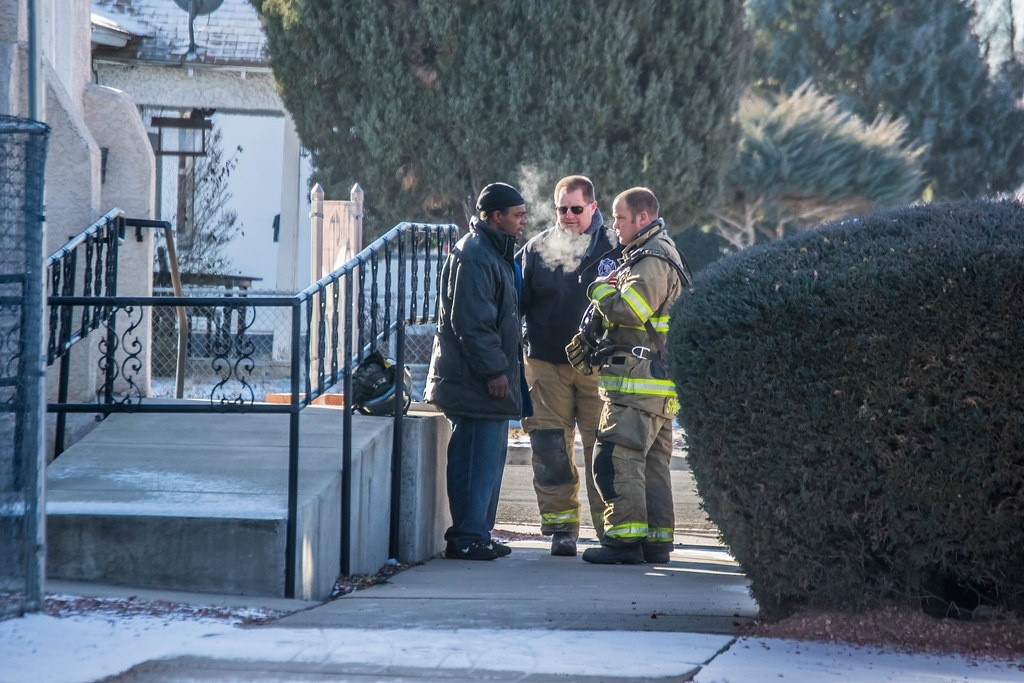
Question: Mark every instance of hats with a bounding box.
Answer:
[475,182,524,211]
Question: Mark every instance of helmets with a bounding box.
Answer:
[351,349,412,416]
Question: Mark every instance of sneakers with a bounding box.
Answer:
[551,532,577,555]
[483,540,512,556]
[445,542,498,560]
[582,543,644,565]
[642,543,670,563]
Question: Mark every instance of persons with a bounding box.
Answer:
[423,175,685,564]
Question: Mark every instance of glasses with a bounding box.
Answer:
[555,199,597,214]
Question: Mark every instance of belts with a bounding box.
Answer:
[604,356,626,365]
